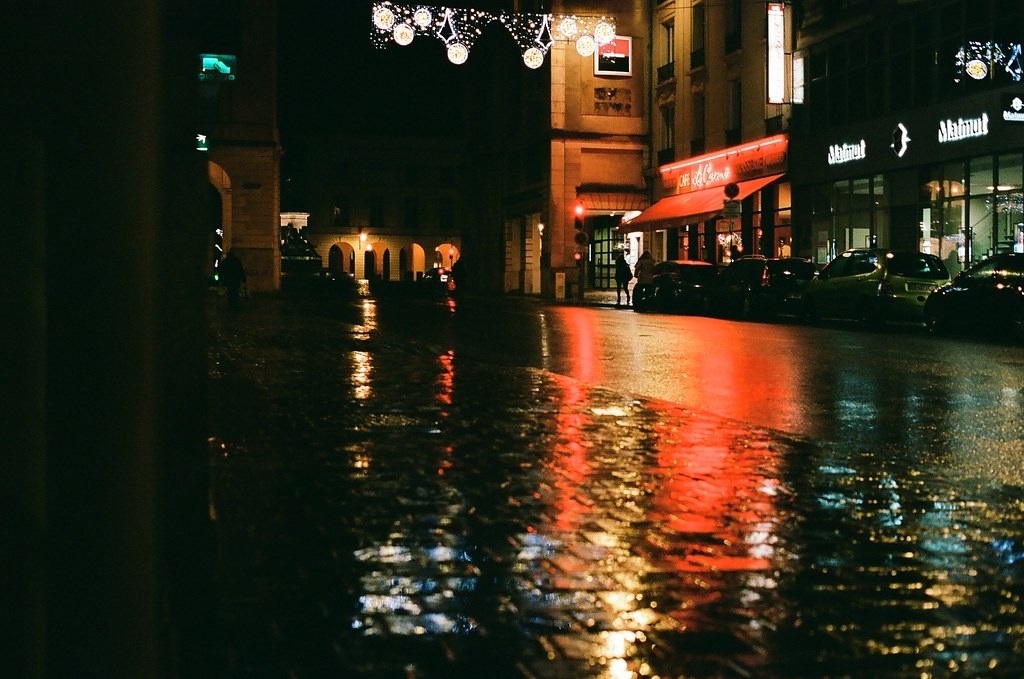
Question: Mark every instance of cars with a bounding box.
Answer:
[633,259,728,313]
[801,248,952,330]
[923,252,1024,335]
[709,255,822,323]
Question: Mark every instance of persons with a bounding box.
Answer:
[634,250,656,282]
[777,238,791,258]
[220,249,247,309]
[615,255,632,305]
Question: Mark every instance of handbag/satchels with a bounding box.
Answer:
[634,258,643,279]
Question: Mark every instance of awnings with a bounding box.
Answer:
[617,173,788,234]
